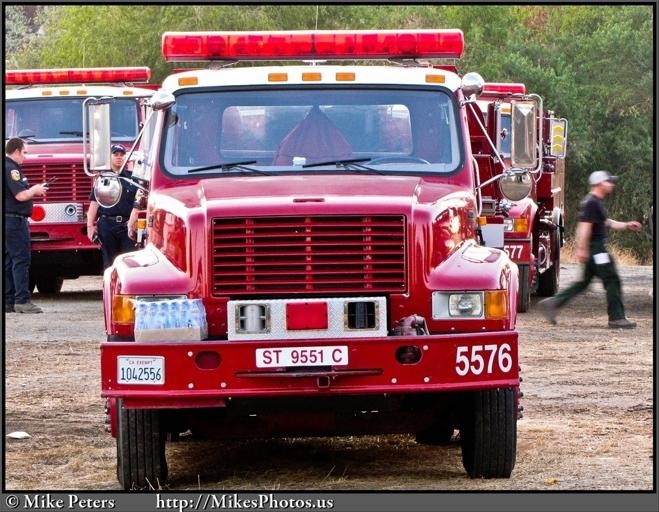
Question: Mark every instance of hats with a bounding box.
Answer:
[111,143,126,153]
[588,170,619,185]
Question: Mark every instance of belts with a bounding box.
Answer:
[5,213,28,219]
[100,213,130,223]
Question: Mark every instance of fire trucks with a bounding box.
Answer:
[83,27,545,492]
[2,66,162,296]
[460,82,568,312]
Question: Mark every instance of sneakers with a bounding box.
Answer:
[536,297,562,326]
[608,316,637,329]
[5,300,44,314]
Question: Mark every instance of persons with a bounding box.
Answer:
[534,170,642,329]
[128,179,150,241]
[87,145,139,271]
[4,137,50,313]
